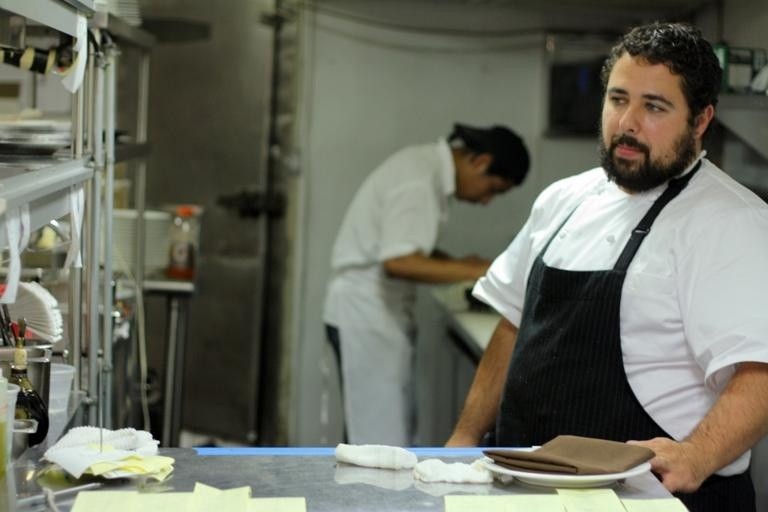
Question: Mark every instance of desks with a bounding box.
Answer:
[3,264,205,448]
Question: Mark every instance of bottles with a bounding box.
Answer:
[165,206,198,283]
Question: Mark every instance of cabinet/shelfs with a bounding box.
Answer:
[441,331,508,449]
[1,1,153,512]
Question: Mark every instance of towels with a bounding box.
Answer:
[480,436,655,476]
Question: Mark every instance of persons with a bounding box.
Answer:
[439,20,767,511]
[322,123,532,448]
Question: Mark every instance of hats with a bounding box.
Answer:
[453,122,529,186]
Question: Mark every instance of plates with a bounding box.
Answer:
[480,455,652,489]
